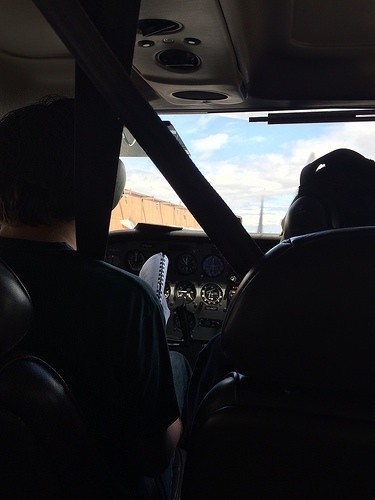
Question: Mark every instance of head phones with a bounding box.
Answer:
[112,159,126,210]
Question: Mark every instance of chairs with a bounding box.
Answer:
[0,264,110,500]
[172,221,375,500]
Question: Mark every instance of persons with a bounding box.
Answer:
[0,101,183,500]
[184,149,375,436]
